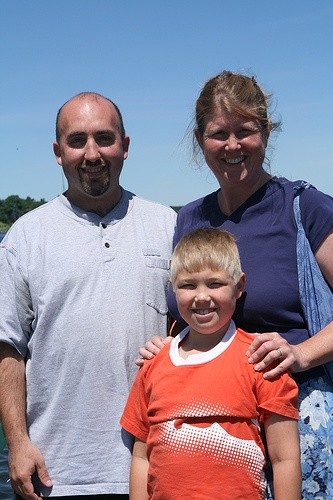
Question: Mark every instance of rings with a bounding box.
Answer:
[277,349,284,358]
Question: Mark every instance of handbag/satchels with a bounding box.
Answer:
[291,177,333,383]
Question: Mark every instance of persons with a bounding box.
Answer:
[134,71,331,500]
[119,224,304,500]
[0,88,181,500]
